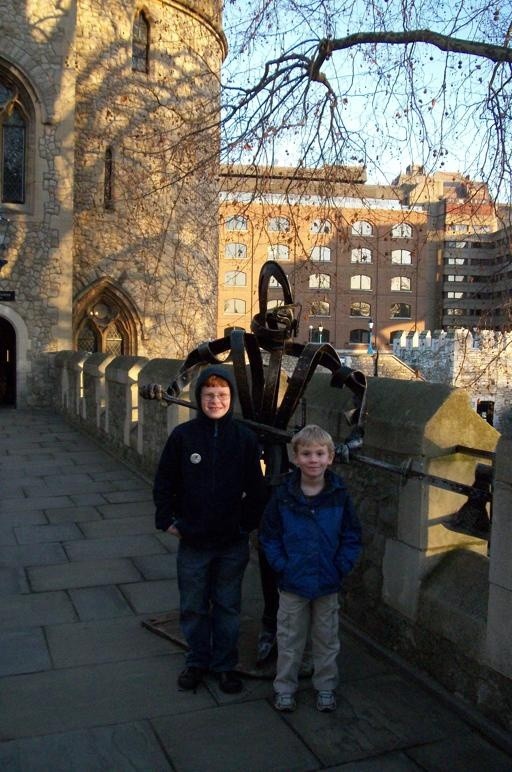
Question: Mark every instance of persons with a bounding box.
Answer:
[258,423,363,715]
[151,365,263,695]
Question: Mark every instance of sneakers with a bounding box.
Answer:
[215,670,246,699]
[272,690,300,713]
[314,689,339,714]
[175,664,207,694]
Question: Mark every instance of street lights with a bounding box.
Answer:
[308,324,313,342]
[318,322,325,344]
[366,317,375,356]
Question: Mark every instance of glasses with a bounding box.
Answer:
[200,392,230,401]
